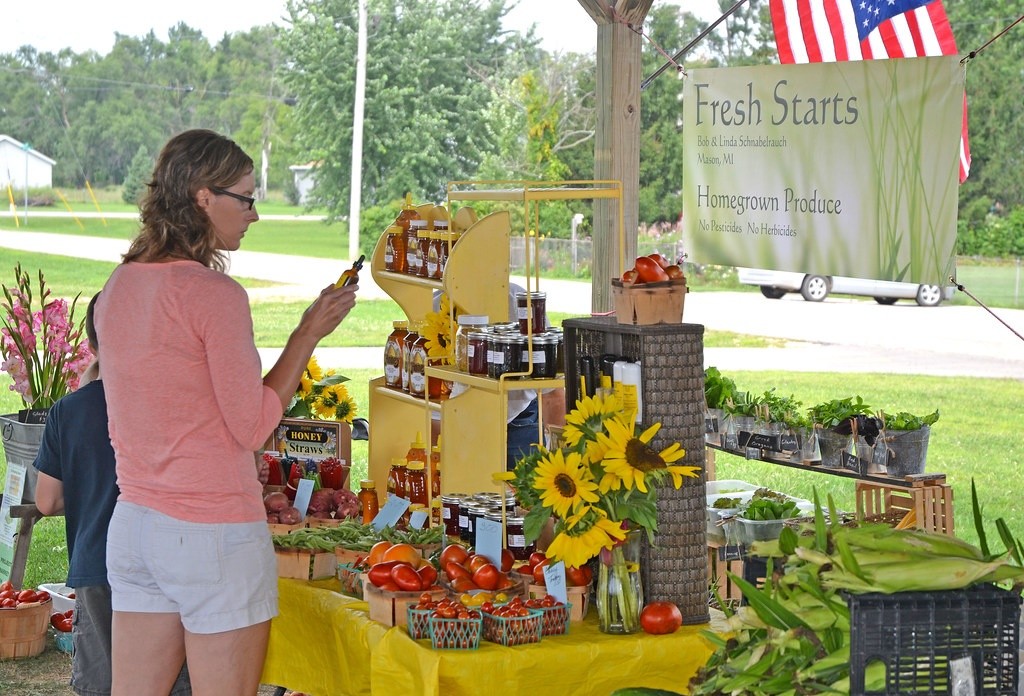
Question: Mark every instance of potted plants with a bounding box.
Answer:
[704,365,939,480]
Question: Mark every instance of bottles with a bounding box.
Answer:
[383,192,564,398]
[333,255,365,289]
[358,431,536,561]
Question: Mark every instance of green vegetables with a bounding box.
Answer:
[709,488,801,522]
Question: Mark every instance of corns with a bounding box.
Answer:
[689,521,1024,696]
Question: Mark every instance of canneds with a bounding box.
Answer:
[455,291,565,381]
[440,491,537,561]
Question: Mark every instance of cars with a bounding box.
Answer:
[734,265,956,308]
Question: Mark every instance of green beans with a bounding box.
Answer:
[272,515,445,579]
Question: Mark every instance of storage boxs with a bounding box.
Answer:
[829,588,1024,696]
[39,582,78,619]
[705,481,845,546]
[611,277,687,325]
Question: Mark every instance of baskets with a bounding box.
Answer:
[266,514,369,599]
[406,594,573,650]
[51,626,75,653]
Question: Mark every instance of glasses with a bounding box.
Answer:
[207,185,255,210]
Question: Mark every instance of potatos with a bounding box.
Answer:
[263,488,360,524]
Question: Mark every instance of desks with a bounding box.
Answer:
[255,576,752,696]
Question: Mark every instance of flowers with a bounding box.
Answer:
[492,389,703,634]
[285,354,371,443]
[1,261,94,418]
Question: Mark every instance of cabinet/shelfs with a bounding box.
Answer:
[370,199,511,510]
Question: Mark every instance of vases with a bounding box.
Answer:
[596,528,644,633]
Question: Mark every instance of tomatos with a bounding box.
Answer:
[368,540,590,649]
[623,254,685,284]
[0,581,50,608]
[640,601,682,634]
[51,609,74,632]
[68,593,75,599]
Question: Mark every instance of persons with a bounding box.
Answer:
[32,291,193,696]
[94,129,359,696]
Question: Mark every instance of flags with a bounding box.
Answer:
[769,1,971,188]
